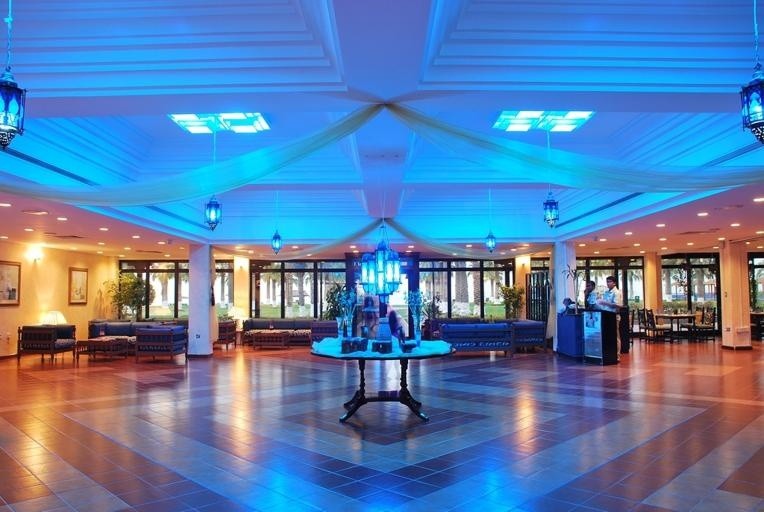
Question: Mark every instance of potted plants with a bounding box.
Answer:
[340,286,363,339]
[324,282,346,340]
[405,288,424,346]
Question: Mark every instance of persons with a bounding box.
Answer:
[597,277,623,361]
[584,281,601,310]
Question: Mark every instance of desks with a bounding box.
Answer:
[309,333,458,424]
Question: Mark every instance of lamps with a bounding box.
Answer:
[357,226,403,319]
[269,173,284,255]
[543,129,559,229]
[0,0,27,152]
[203,118,223,231]
[740,1,763,145]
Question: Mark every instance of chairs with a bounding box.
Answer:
[628,306,716,345]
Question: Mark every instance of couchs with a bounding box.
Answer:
[219,316,313,352]
[17,317,187,365]
[420,312,544,357]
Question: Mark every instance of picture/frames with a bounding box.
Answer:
[68,265,88,306]
[0,259,22,308]
